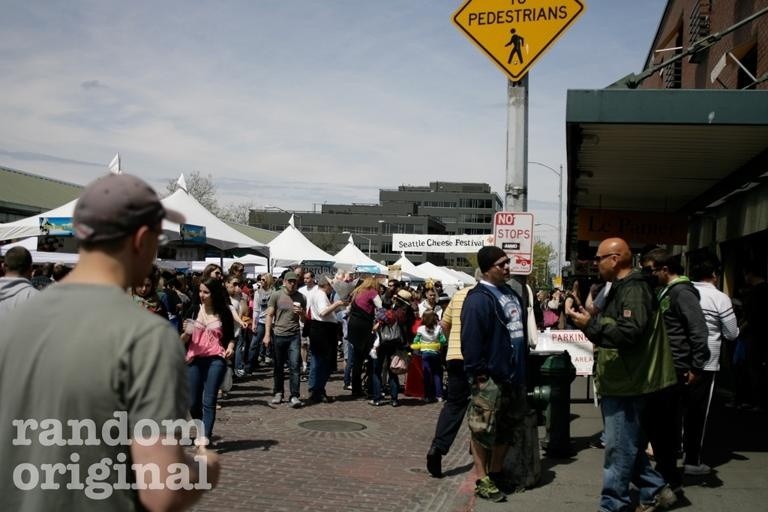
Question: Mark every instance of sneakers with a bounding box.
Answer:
[591,439,607,450]
[634,483,679,512]
[684,463,712,476]
[391,399,398,407]
[272,393,283,404]
[368,399,383,406]
[426,446,442,477]
[291,397,301,408]
[474,476,504,503]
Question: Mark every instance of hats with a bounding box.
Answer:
[285,271,298,281]
[74,173,186,242]
[477,246,507,273]
[393,289,412,306]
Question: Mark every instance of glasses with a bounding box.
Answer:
[594,252,623,264]
[233,282,241,287]
[493,258,510,267]
[215,271,221,275]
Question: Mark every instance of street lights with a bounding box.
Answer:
[342,230,371,258]
[249,207,268,227]
[377,220,403,233]
[263,204,303,233]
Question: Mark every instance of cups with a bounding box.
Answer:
[185,319,195,334]
[293,301,300,307]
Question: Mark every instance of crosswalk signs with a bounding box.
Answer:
[451,0,585,83]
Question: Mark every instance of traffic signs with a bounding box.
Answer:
[492,212,535,276]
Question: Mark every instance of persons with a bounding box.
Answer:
[0,173,768,512]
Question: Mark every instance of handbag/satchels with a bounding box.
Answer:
[390,355,409,375]
[543,310,560,327]
[219,367,233,392]
[380,321,402,342]
